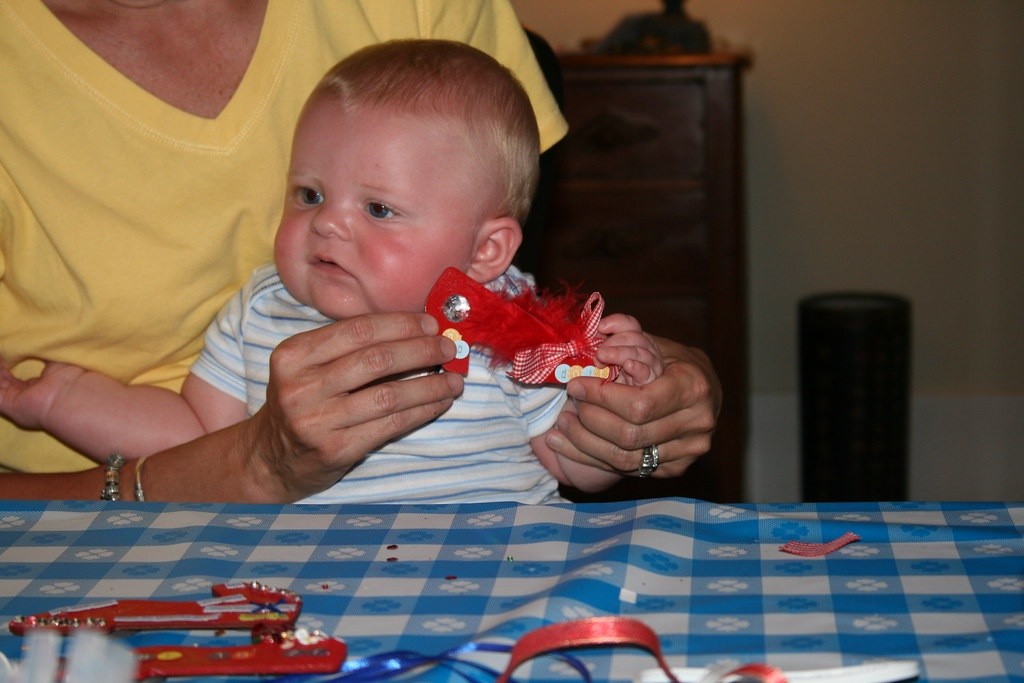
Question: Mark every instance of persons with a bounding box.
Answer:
[0,38,664,506]
[0,0,723,504]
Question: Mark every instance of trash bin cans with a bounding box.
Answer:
[800,291,912,503]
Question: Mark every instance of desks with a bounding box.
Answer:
[0,488,1023,683]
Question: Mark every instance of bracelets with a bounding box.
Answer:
[100,454,126,501]
[136,455,149,501]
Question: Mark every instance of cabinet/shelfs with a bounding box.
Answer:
[514,58,753,500]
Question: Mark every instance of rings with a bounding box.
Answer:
[639,446,660,477]
